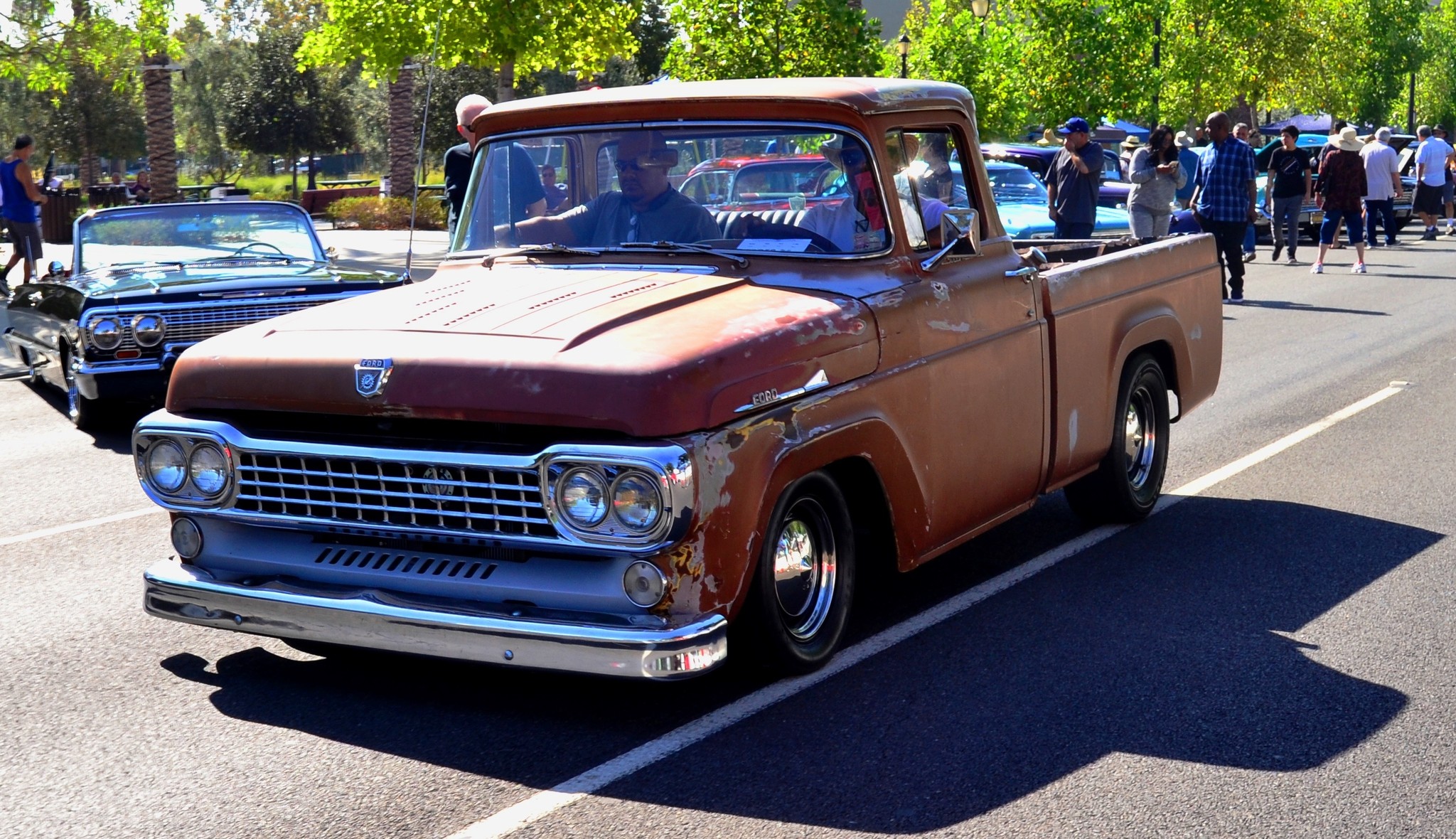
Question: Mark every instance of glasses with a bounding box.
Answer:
[842,151,866,167]
[542,173,554,177]
[613,159,646,173]
[461,124,475,133]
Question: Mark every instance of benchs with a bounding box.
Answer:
[706,209,810,241]
[184,198,224,201]
[299,186,380,229]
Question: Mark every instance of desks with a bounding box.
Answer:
[178,184,220,197]
[416,184,446,196]
[315,179,377,188]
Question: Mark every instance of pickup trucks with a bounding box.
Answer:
[129,73,1222,687]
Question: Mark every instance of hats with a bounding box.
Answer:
[1036,117,1449,149]
[818,132,919,177]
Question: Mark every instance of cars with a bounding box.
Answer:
[1188,134,1346,243]
[289,157,322,172]
[820,160,1134,249]
[679,154,852,212]
[2,11,442,425]
[1354,133,1419,230]
[1407,141,1456,219]
[949,142,1201,236]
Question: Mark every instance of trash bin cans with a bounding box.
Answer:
[89,185,127,207]
[40,186,82,243]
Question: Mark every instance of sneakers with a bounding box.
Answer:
[1217,224,1456,304]
[0,277,11,297]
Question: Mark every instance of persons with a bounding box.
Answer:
[797,131,953,253]
[1042,118,1104,238]
[1127,125,1188,240]
[1196,118,1456,273]
[916,132,953,208]
[439,93,549,252]
[539,164,569,212]
[489,128,724,249]
[1173,131,1200,210]
[1118,134,1139,181]
[0,134,55,297]
[35,167,152,207]
